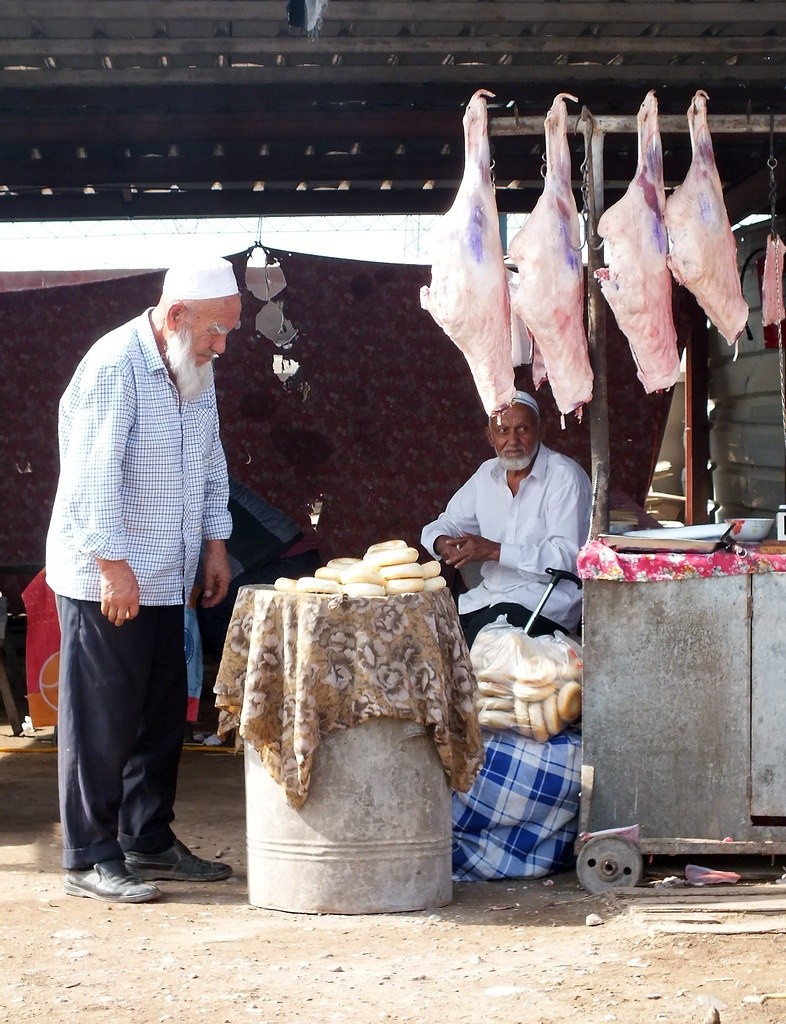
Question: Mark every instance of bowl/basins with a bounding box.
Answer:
[724,519,775,545]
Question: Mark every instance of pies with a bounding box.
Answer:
[274,539,447,598]
[470,635,582,744]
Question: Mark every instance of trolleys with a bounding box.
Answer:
[477,113,785,898]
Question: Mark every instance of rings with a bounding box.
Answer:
[456,544,461,553]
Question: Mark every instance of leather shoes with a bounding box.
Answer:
[123,839,232,883]
[64,857,162,903]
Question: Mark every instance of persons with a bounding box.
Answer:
[418,388,597,659]
[39,251,245,906]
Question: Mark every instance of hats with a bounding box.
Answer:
[161,256,240,301]
[515,391,542,420]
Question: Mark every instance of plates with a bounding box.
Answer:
[597,533,722,554]
[621,522,732,542]
